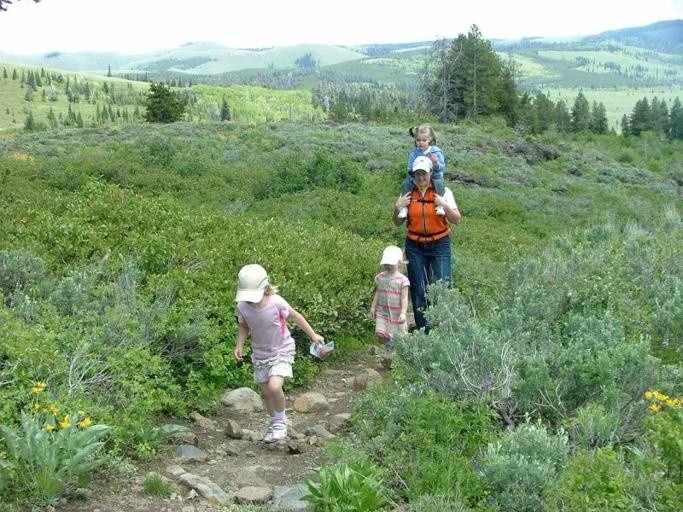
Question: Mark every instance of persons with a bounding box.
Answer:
[397,124,445,219]
[370,246,410,344]
[391,155,460,333]
[233,264,324,444]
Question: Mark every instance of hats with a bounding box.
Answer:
[234,262,268,304]
[379,243,403,267]
[411,156,433,173]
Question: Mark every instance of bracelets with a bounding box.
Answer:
[393,209,398,216]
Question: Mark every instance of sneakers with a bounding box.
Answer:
[386,336,395,351]
[398,207,410,220]
[273,417,287,439]
[264,424,279,443]
[435,206,447,217]
